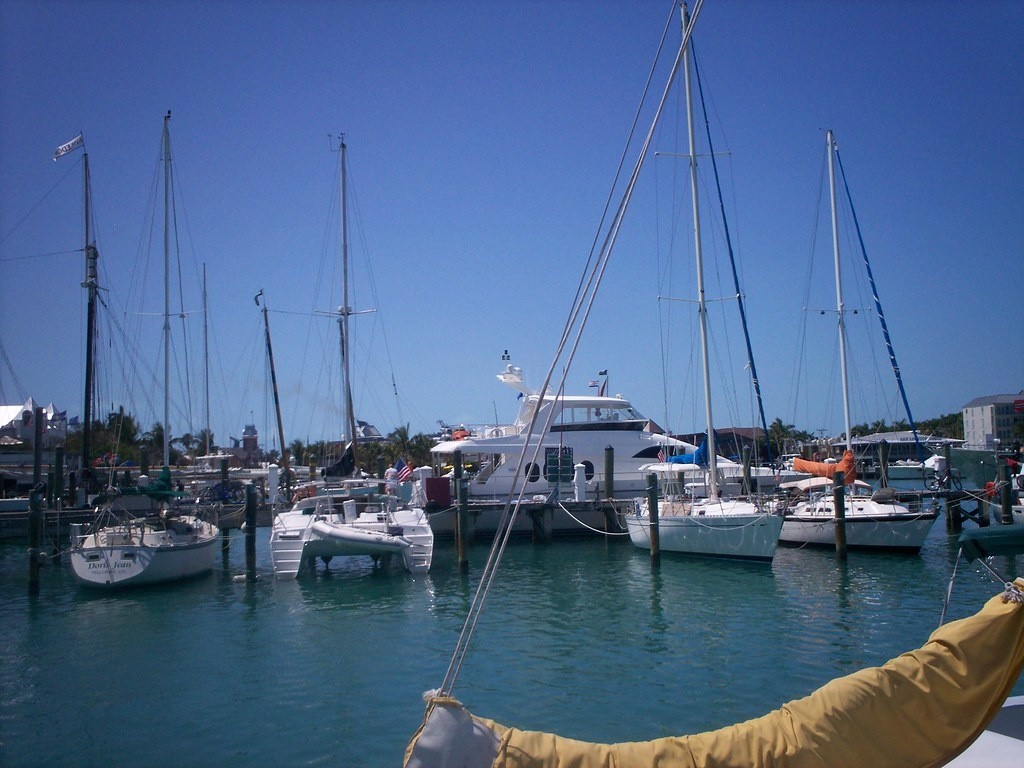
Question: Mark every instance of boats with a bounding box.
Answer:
[424,365,814,531]
[922,433,1024,500]
[992,453,1024,523]
[779,454,801,470]
[875,458,934,478]
[269,479,434,578]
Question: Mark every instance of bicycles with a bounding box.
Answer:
[197,477,264,505]
[924,467,962,491]
[278,483,309,501]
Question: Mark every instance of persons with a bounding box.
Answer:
[384,464,400,497]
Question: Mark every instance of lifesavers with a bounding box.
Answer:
[985,482,995,496]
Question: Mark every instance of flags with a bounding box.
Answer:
[356,419,382,437]
[599,369,608,375]
[589,379,599,387]
[517,392,523,401]
[393,455,411,483]
[657,448,664,462]
[773,471,780,482]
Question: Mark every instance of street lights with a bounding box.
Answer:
[818,428,827,462]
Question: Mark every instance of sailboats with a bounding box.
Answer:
[69,110,220,587]
[625,1,789,561]
[752,129,943,553]
